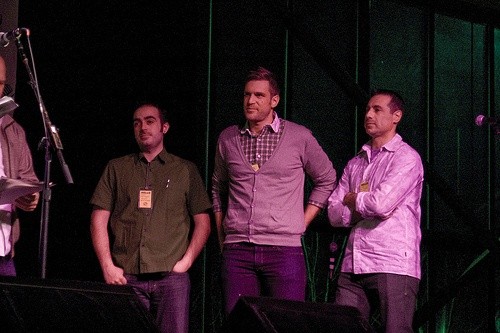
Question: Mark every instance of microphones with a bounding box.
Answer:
[329,242,338,280]
[475,115,500,126]
[0,28,28,48]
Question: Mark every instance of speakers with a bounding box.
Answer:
[219,296,371,333]
[0,274,162,333]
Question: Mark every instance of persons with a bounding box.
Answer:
[89,99,213,333]
[212,66,336,315]
[327,88,425,333]
[0,54,40,277]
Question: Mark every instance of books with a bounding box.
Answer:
[0,176,56,205]
